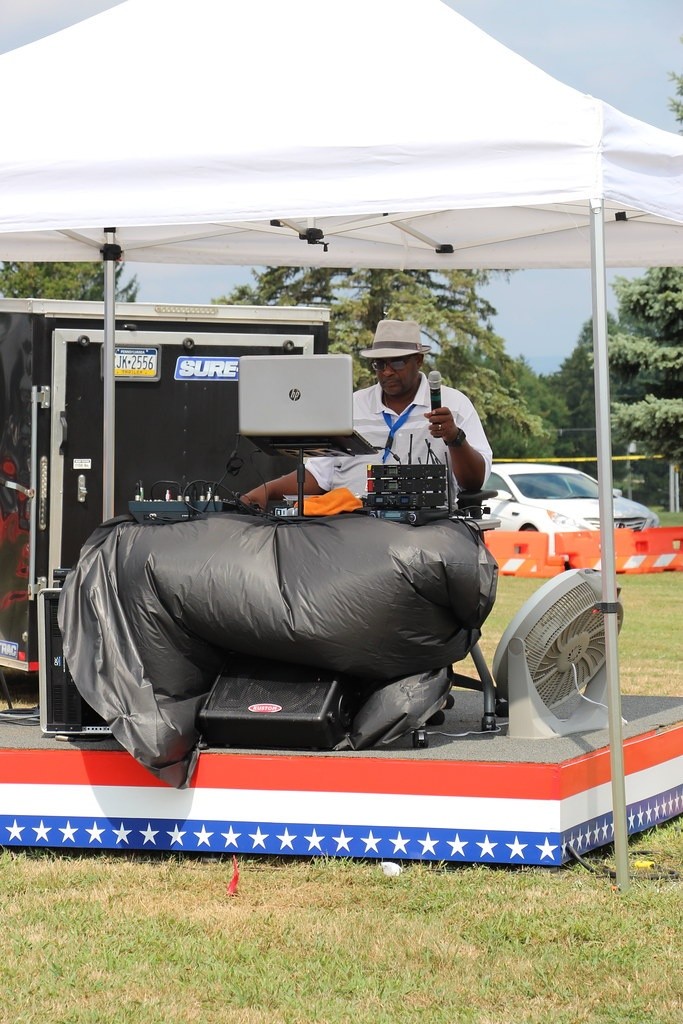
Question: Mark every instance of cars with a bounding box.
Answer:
[479,462,660,562]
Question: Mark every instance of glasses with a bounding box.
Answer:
[371,354,417,371]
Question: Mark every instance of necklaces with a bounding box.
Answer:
[384,394,388,407]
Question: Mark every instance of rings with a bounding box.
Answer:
[439,424,442,430]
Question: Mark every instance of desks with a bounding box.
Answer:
[409,520,502,748]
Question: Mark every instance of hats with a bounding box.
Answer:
[360,320,432,358]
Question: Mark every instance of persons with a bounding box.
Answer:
[241,320,493,509]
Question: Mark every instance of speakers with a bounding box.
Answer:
[32,586,110,736]
[194,650,357,752]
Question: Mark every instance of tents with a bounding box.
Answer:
[0,0,682,892]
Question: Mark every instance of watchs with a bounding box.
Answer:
[444,427,466,447]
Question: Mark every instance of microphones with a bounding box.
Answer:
[428,370,443,438]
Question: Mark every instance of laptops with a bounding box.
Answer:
[238,355,380,455]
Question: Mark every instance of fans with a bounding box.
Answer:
[492,568,624,741]
